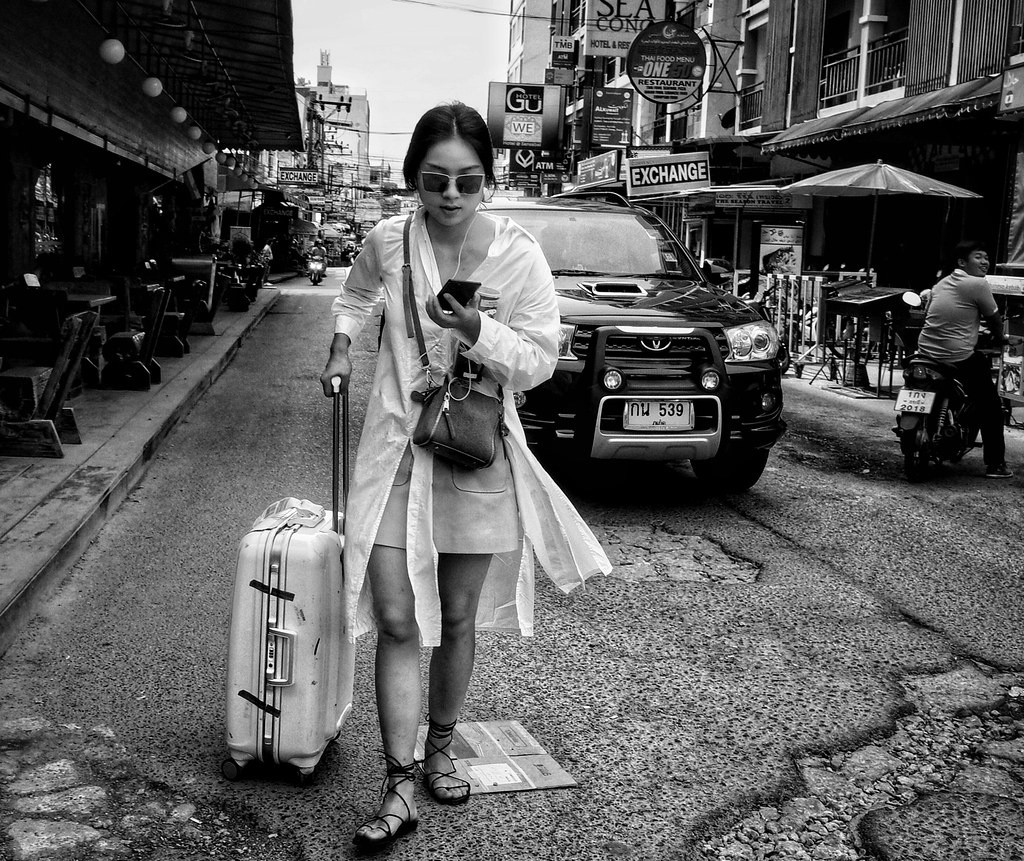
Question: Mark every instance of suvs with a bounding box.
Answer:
[470,191,788,491]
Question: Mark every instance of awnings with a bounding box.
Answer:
[0,0,307,151]
[763,73,1001,156]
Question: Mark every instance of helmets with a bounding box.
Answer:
[317,239,323,243]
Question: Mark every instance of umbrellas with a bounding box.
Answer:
[776,159,984,389]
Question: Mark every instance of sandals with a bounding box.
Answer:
[422,713,471,805]
[352,749,419,849]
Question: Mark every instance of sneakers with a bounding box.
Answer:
[986,462,1014,477]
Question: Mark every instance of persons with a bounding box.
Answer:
[259,236,326,286]
[320,101,559,856]
[917,238,1014,478]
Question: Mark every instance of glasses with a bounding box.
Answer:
[419,168,485,194]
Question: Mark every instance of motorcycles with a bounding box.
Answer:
[892,291,1023,485]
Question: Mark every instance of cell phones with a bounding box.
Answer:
[437,278,481,311]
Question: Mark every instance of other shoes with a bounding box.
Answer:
[264,281,273,285]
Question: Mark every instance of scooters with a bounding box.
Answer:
[308,256,326,285]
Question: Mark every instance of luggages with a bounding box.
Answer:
[222,375,350,785]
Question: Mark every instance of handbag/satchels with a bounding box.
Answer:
[410,371,504,468]
